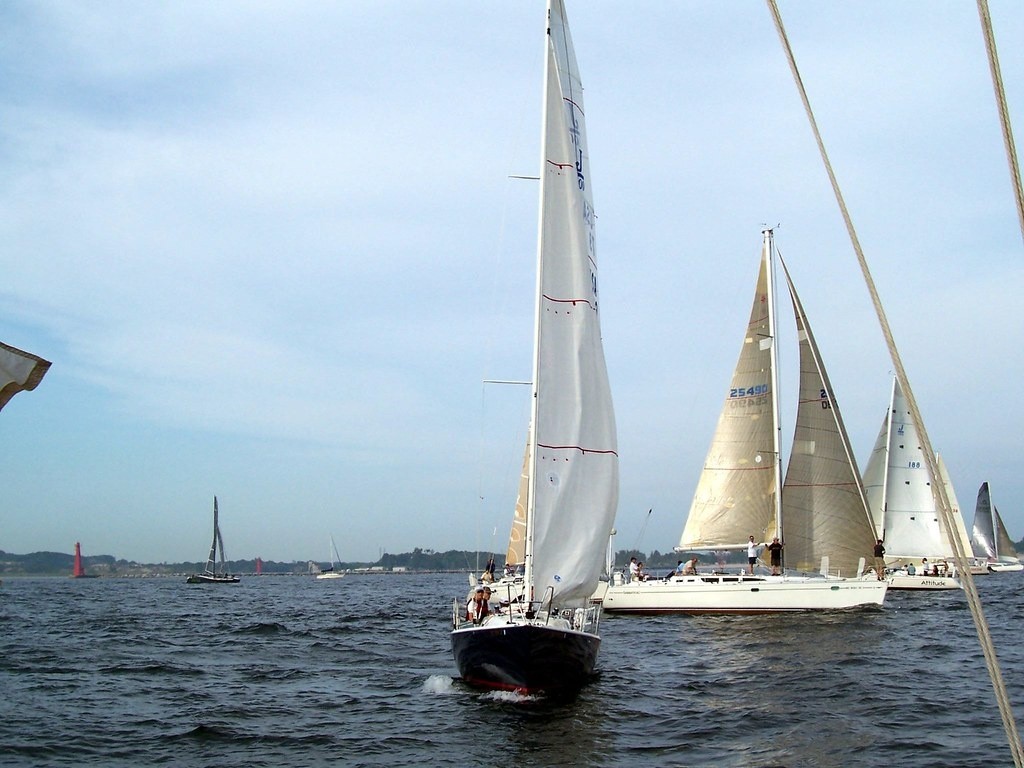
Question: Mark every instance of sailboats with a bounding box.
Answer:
[603,222,890,614]
[862,378,1024,590]
[316,536,346,579]
[74,542,101,578]
[186,495,241,584]
[451,1,620,710]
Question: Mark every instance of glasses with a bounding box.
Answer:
[477,590,483,593]
[486,593,491,594]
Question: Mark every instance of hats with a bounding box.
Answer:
[475,586,484,593]
[878,540,883,543]
[483,587,492,592]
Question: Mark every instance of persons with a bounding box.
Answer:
[486,558,496,581]
[504,562,525,577]
[874,539,886,580]
[629,557,644,581]
[907,563,916,575]
[481,569,492,585]
[932,565,938,575]
[494,605,504,613]
[675,560,685,576]
[747,535,759,575]
[682,557,697,575]
[765,537,783,576]
[922,558,929,576]
[974,559,978,567]
[902,565,908,571]
[466,586,493,625]
[943,561,949,573]
